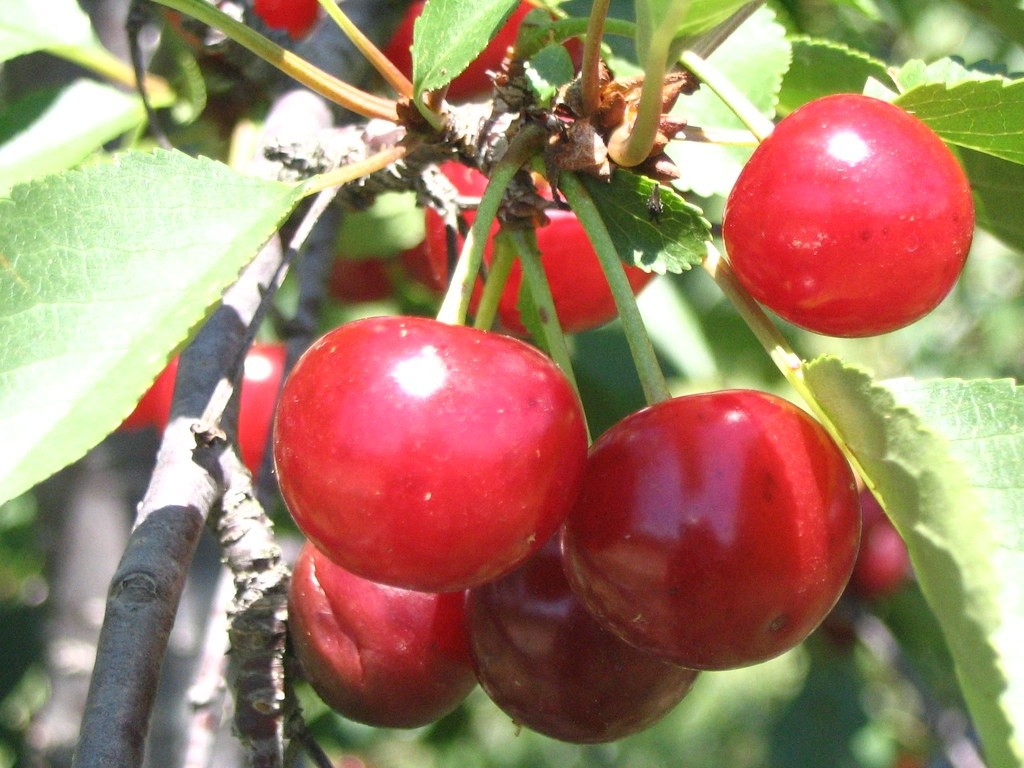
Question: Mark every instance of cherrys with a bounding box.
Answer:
[117,0,976,746]
